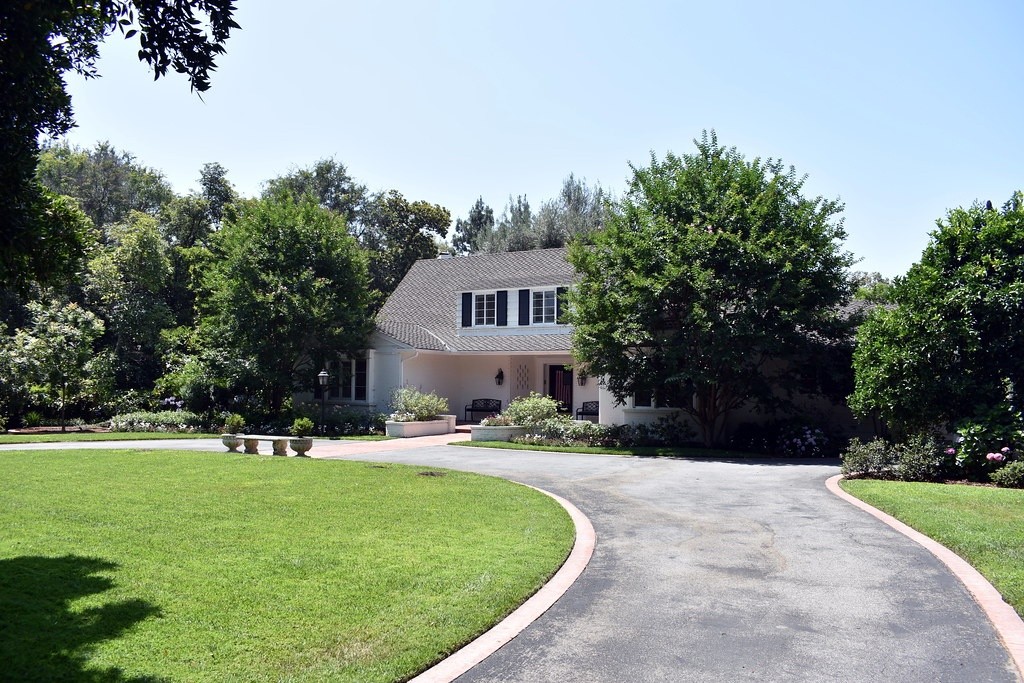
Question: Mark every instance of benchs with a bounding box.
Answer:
[238,437,305,457]
[465,398,501,422]
[575,401,599,420]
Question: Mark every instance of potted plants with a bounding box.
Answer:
[470,415,525,441]
[221,413,246,453]
[495,368,504,385]
[385,384,449,437]
[576,368,587,386]
[288,417,314,457]
